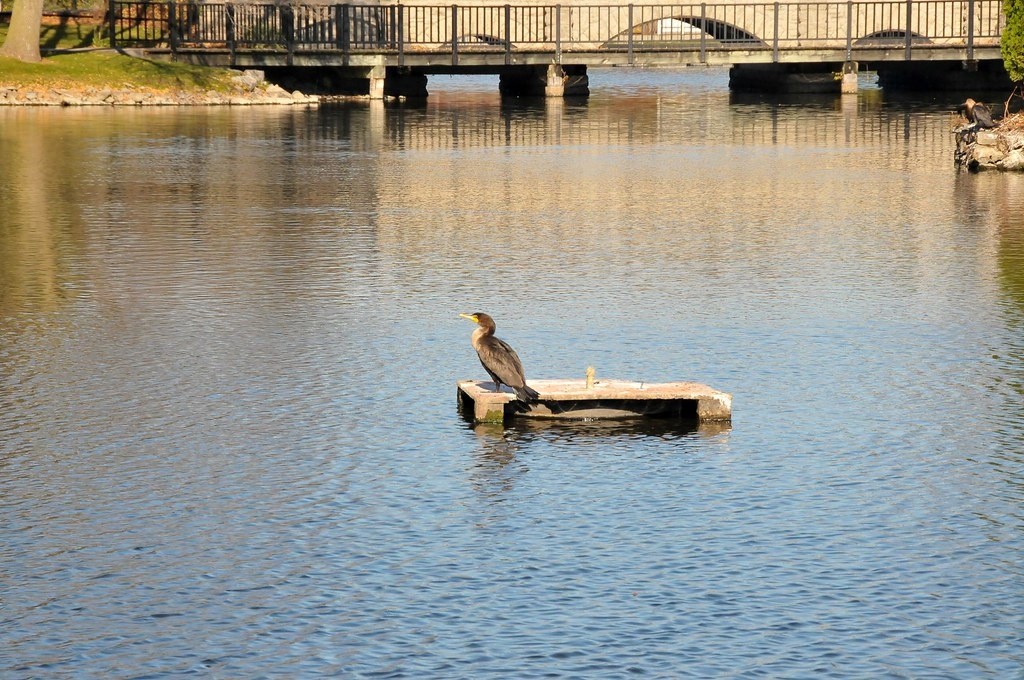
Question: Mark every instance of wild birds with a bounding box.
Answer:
[960,98,997,128]
[459,312,540,403]
